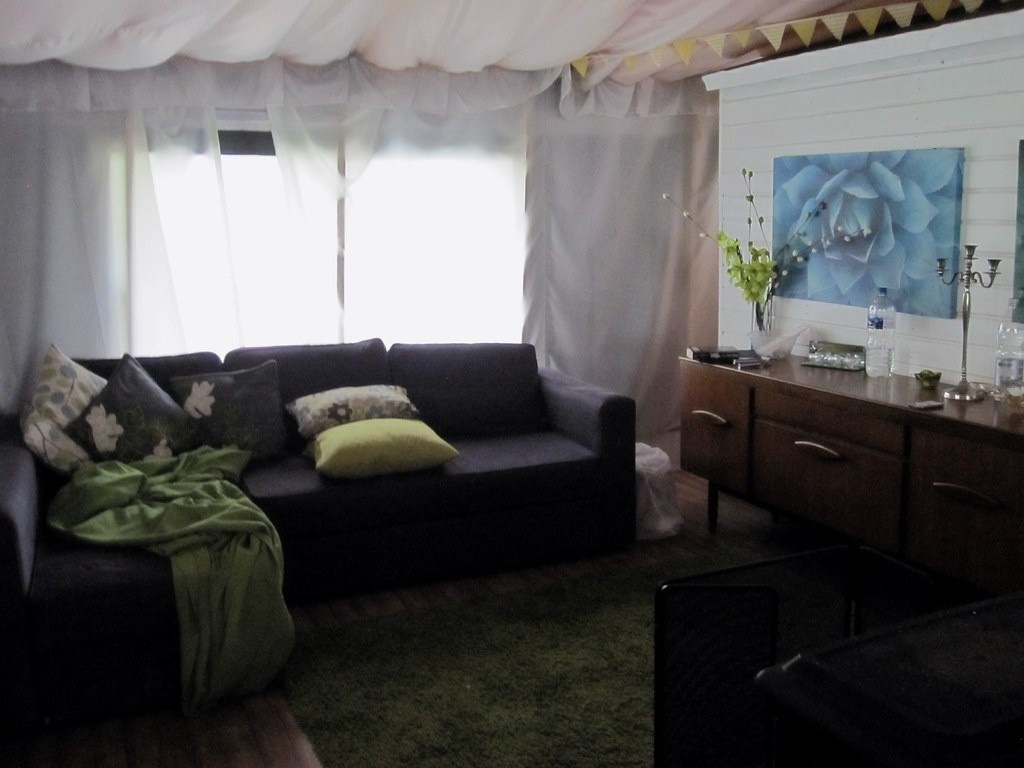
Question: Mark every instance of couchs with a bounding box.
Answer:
[0,338,638,731]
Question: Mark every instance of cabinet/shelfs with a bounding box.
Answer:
[678,355,1024,612]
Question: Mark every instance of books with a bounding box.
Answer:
[687,345,740,360]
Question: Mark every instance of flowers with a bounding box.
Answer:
[662,168,863,332]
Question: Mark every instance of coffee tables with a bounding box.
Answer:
[652,545,1024,768]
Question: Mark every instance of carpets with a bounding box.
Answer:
[281,546,750,768]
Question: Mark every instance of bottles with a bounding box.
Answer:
[865,286,896,379]
[994,298,1024,410]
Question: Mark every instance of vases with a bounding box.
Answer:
[751,296,772,350]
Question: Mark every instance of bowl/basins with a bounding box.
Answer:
[749,331,799,359]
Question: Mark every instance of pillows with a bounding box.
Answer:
[286,385,420,438]
[21,343,108,471]
[65,353,185,463]
[302,419,461,482]
[169,359,286,461]
